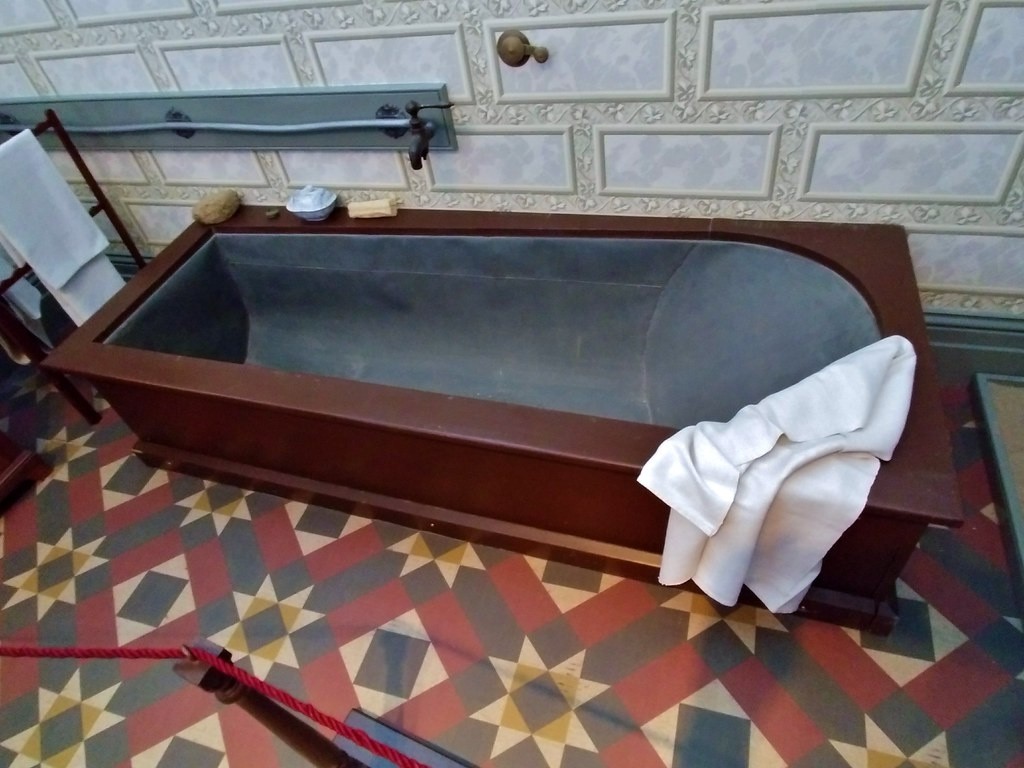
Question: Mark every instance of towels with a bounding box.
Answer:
[1,258,54,366]
[637,336,917,613]
[0,129,127,327]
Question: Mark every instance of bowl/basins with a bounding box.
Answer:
[285,192,338,222]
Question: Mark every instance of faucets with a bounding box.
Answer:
[404,99,455,170]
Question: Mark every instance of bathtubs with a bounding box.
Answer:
[39,205,965,636]
